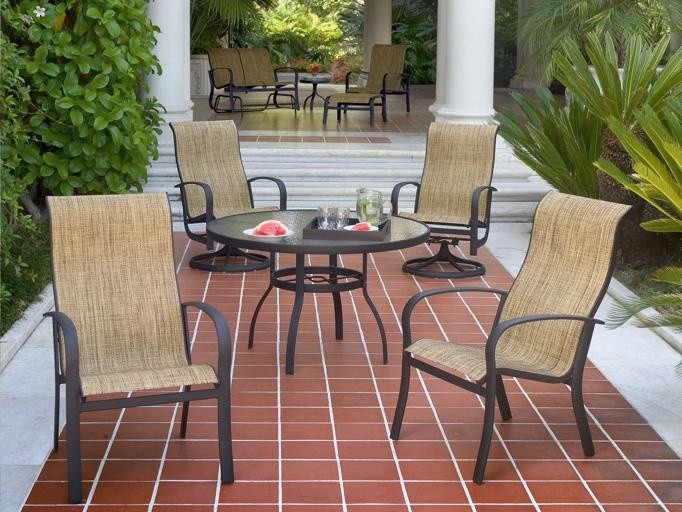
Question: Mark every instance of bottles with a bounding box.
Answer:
[317,205,350,231]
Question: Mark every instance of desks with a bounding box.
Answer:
[297,76,332,110]
[206,208,431,377]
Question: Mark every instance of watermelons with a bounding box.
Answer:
[350,222,372,230]
[252,220,288,237]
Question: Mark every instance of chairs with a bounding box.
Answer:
[321,44,412,127]
[169,120,286,274]
[387,189,633,484]
[389,123,499,280]
[44,194,236,505]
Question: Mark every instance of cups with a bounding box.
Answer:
[355,187,393,226]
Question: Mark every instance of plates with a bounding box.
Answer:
[343,225,379,231]
[242,229,294,241]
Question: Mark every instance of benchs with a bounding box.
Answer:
[208,48,301,116]
